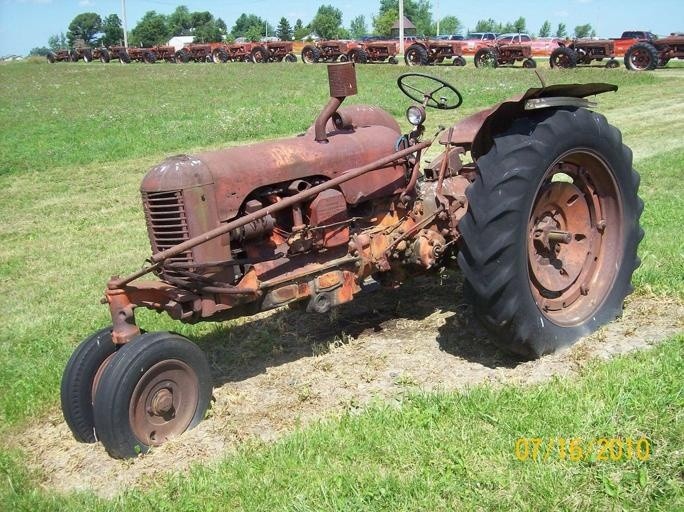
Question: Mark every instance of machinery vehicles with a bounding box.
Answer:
[60,61,645,465]
